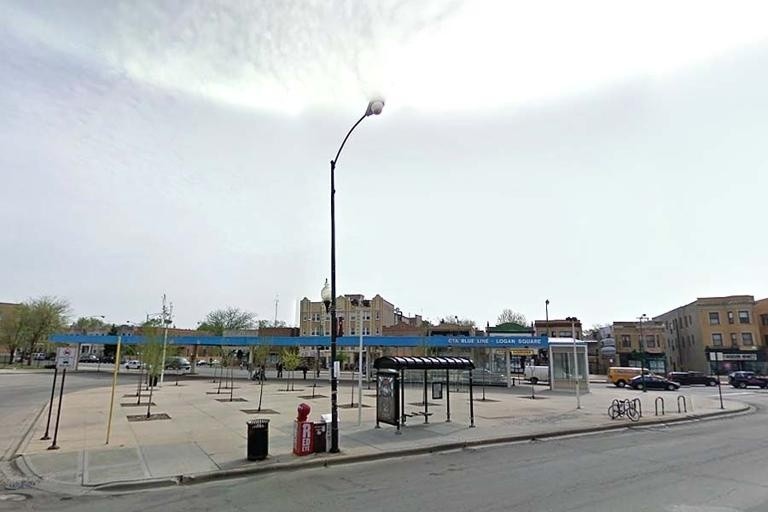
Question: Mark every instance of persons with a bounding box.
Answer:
[277,363,282,378]
[301,362,307,380]
[275,360,279,378]
[239,360,244,370]
[251,370,259,380]
[260,361,266,381]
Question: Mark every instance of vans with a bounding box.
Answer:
[605,366,653,389]
[524,366,550,385]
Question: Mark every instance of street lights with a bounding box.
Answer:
[636,312,647,367]
[530,320,533,336]
[486,320,490,336]
[544,299,551,337]
[454,315,460,335]
[79,315,105,336]
[347,296,374,427]
[316,92,385,459]
[124,319,141,369]
[273,292,280,328]
[146,312,170,323]
[159,319,173,387]
[563,316,583,410]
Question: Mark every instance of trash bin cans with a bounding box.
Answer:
[150,375,158,386]
[246,418,270,461]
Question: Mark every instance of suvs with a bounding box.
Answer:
[724,370,767,390]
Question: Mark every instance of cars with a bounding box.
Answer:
[625,373,680,391]
[13,351,223,371]
[350,359,507,385]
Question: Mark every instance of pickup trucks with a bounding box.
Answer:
[666,370,720,388]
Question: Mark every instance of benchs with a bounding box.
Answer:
[402,411,433,426]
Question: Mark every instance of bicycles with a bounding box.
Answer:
[604,400,640,423]
